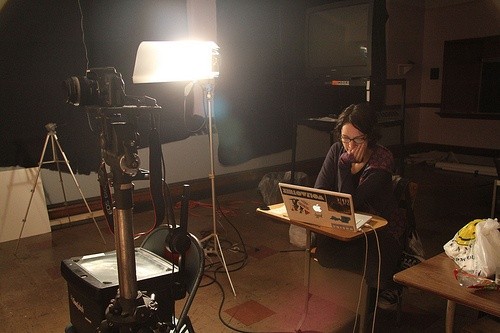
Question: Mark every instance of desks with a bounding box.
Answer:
[256,201,388,333]
[392,251,500,333]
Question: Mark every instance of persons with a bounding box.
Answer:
[315,103,406,333]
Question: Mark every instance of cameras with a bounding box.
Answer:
[64,66,125,107]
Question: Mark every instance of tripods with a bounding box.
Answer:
[14,123,110,258]
[200,87,237,296]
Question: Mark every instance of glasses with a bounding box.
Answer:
[340,136,369,144]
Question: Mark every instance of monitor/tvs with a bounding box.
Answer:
[305,0,387,79]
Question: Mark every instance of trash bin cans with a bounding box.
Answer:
[267,171,307,206]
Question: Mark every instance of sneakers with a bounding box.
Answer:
[377,287,404,310]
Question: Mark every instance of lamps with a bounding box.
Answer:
[131,40,224,84]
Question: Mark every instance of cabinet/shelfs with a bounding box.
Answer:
[290,78,407,184]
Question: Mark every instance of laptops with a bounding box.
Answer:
[278,182,373,232]
